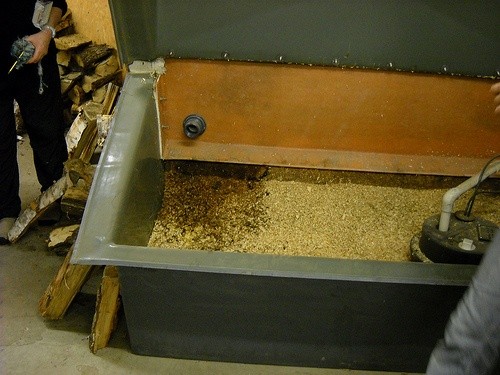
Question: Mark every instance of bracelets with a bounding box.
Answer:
[45,25,56,38]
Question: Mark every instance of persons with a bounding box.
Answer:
[0,0,68,242]
